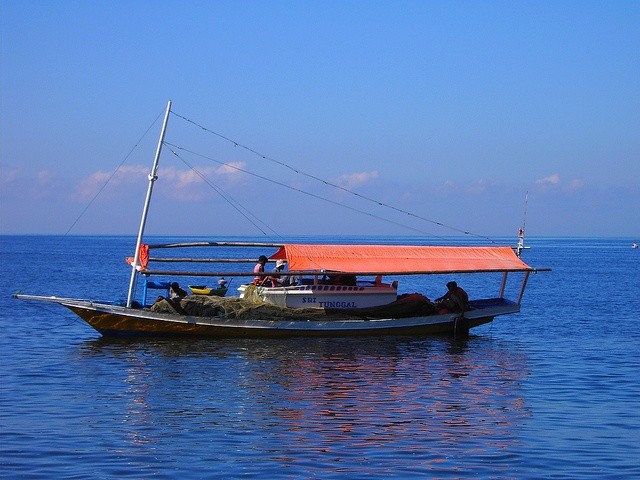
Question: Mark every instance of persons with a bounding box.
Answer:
[268,259,299,286]
[434,280,468,312]
[154,281,189,306]
[253,255,288,287]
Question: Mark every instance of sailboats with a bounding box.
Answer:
[12,100,555,337]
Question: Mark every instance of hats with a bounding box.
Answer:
[275,260,285,268]
